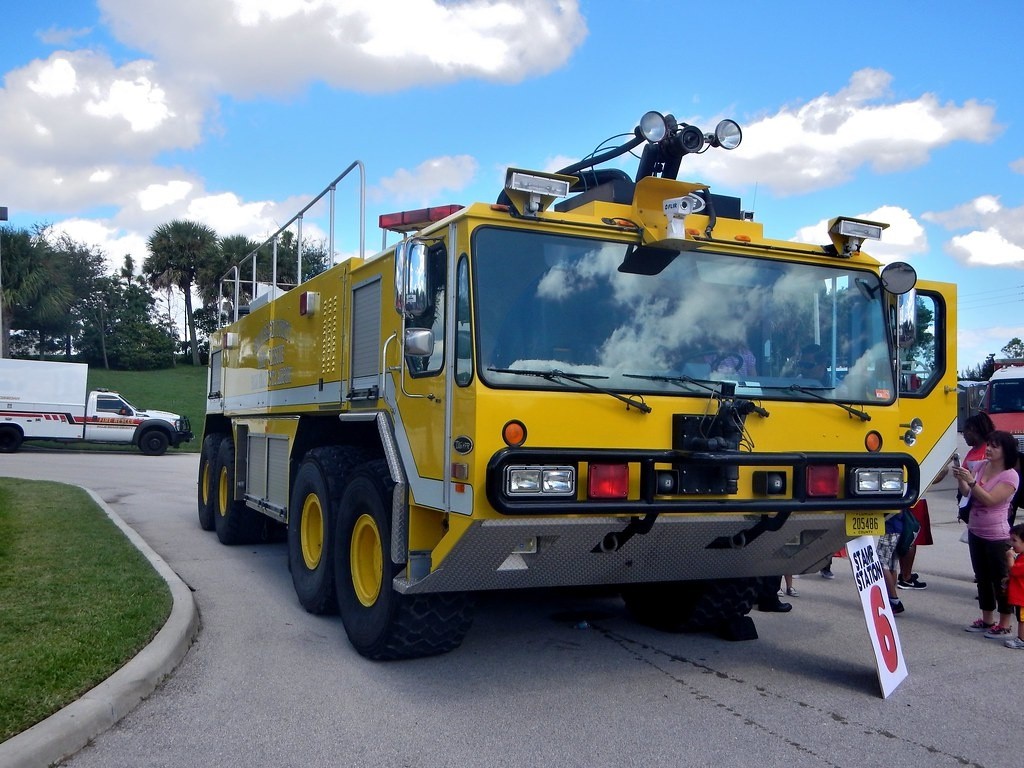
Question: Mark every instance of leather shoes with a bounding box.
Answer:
[759,600,792,612]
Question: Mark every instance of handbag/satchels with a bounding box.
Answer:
[897,508,921,557]
[959,492,971,524]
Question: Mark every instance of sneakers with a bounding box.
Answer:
[889,597,904,615]
[777,589,784,597]
[984,625,1012,638]
[898,574,918,580]
[1005,637,1024,649]
[787,587,799,597]
[965,618,997,633]
[821,570,834,578]
[897,580,927,589]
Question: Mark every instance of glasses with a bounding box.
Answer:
[798,362,821,369]
[961,430,971,435]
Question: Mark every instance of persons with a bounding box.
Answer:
[753,343,1024,650]
[704,318,756,376]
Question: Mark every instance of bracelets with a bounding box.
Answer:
[968,479,976,488]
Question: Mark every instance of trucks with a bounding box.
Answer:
[979,354,1024,456]
[0,357,198,456]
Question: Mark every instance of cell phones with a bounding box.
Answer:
[953,453,962,468]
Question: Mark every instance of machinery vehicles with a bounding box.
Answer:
[194,107,959,659]
